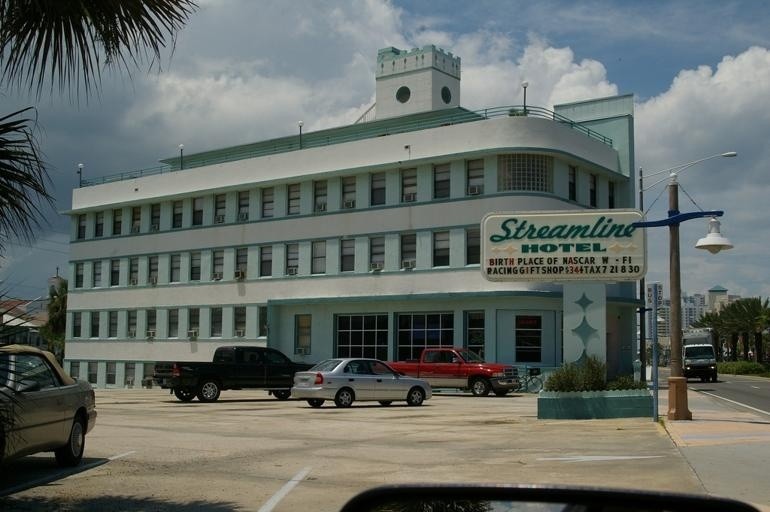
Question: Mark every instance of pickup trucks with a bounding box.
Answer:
[153,346,317,403]
[388,348,521,397]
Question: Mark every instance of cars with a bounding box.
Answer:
[0,343,97,463]
[291,357,432,408]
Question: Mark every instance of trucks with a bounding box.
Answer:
[681,327,717,383]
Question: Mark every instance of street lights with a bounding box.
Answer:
[521,81,529,116]
[179,144,184,169]
[78,163,84,187]
[639,151,737,380]
[298,121,304,149]
[632,173,734,420]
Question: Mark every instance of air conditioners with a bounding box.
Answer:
[403,192,415,202]
[232,270,242,279]
[214,214,224,223]
[187,330,199,337]
[343,199,354,209]
[316,202,326,211]
[150,277,157,284]
[238,212,247,220]
[131,225,140,233]
[467,184,479,195]
[296,347,305,354]
[371,263,381,270]
[130,279,137,285]
[402,260,413,268]
[146,331,154,338]
[127,331,135,337]
[151,224,158,230]
[234,329,244,336]
[211,272,221,280]
[286,268,296,275]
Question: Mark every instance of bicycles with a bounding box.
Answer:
[509,367,543,393]
